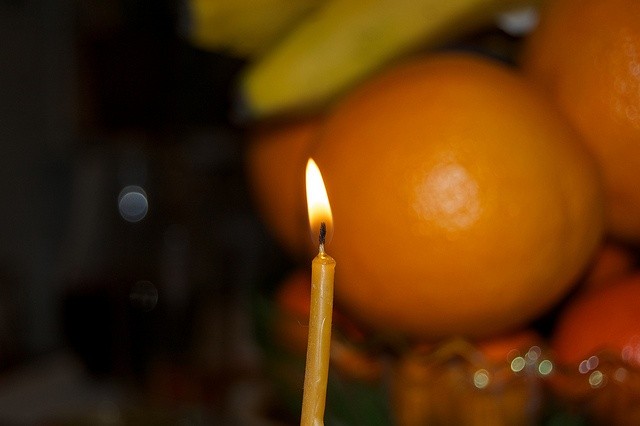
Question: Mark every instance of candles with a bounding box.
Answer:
[299,159,338,426]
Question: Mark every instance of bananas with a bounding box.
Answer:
[189,0,509,123]
